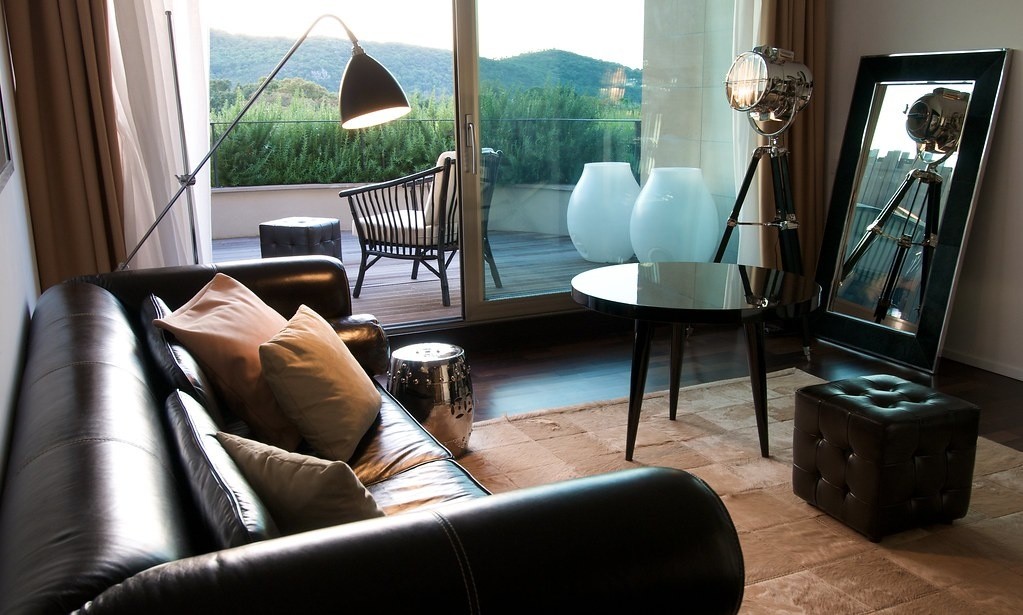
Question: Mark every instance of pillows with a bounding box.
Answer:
[258,303,382,463]
[165,389,280,548]
[140,293,224,428]
[217,431,384,536]
[153,274,301,453]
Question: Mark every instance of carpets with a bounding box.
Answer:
[457,368,1023,615]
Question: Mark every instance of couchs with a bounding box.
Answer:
[0,254,747,615]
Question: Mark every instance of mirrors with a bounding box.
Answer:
[812,47,1014,375]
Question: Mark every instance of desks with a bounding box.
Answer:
[571,262,822,461]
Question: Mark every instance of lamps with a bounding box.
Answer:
[116,14,412,272]
[837,87,972,321]
[684,46,816,364]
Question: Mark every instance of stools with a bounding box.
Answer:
[259,217,343,263]
[793,374,982,543]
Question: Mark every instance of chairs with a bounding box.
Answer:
[337,148,503,306]
